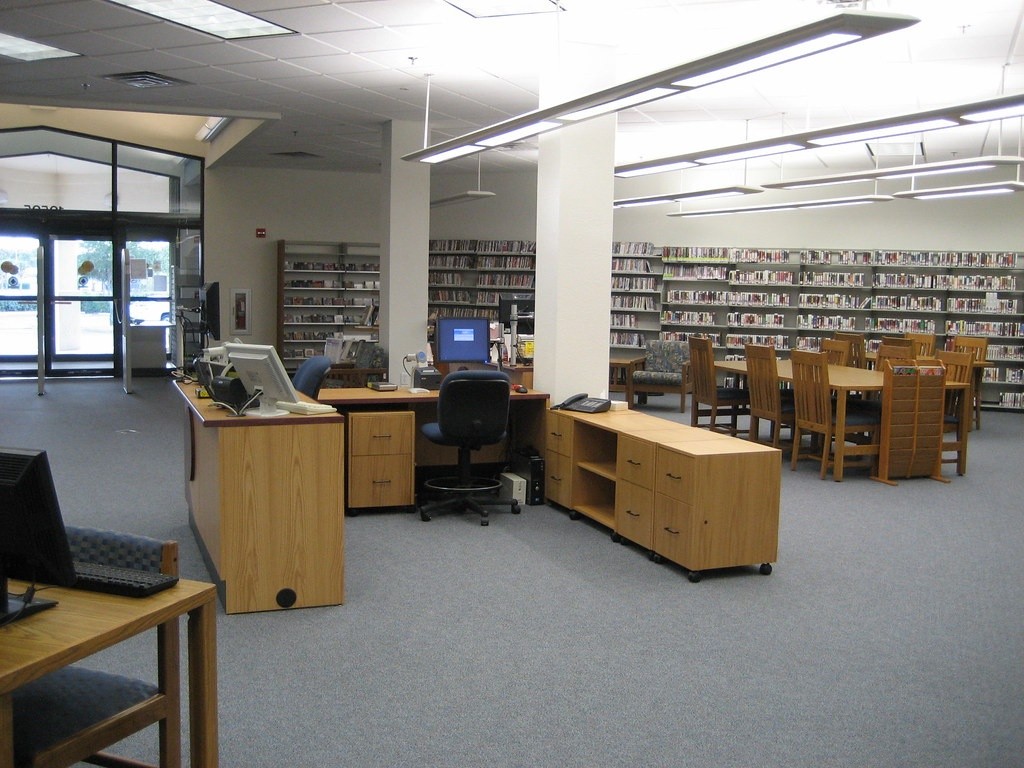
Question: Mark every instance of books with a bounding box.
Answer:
[283,329,343,340]
[871,250,1017,291]
[366,381,398,390]
[284,261,381,271]
[875,292,1024,338]
[283,348,324,358]
[610,276,657,311]
[727,248,794,286]
[794,249,883,353]
[429,239,536,269]
[339,339,365,360]
[955,344,1024,408]
[659,288,790,351]
[612,241,654,272]
[283,306,380,325]
[283,296,380,305]
[283,279,380,289]
[610,314,647,349]
[428,272,535,320]
[662,245,733,280]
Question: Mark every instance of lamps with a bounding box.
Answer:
[398,2,1024,217]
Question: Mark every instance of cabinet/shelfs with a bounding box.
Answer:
[546,407,781,583]
[660,256,1024,412]
[316,388,551,516]
[277,239,666,358]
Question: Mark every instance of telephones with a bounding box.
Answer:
[560,393,612,413]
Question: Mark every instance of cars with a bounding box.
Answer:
[131,300,170,325]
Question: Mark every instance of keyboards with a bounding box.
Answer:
[276,400,338,416]
[70,560,179,598]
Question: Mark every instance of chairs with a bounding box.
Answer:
[688,332,993,482]
[628,341,693,414]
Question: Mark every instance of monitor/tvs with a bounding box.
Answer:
[0,446,78,626]
[498,293,535,335]
[225,343,300,417]
[175,281,221,383]
[437,318,490,363]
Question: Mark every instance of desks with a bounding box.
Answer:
[608,357,633,392]
[0,577,220,768]
[711,361,971,482]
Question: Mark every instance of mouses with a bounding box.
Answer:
[516,387,528,393]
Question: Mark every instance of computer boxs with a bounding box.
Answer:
[511,451,545,506]
